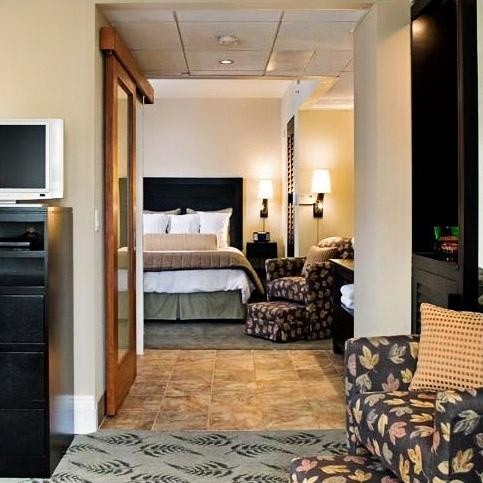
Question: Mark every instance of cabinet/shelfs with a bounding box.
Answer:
[0,207,74,478]
[329,258,354,356]
[410,1,476,339]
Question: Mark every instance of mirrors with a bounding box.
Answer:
[105,54,138,416]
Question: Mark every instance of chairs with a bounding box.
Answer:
[244,237,352,342]
[289,335,483,483]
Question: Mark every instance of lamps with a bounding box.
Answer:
[311,168,332,218]
[258,180,273,231]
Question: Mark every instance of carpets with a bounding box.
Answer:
[143,321,335,350]
[0,427,347,483]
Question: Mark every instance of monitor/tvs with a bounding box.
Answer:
[0,118,66,209]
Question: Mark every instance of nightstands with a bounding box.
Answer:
[246,242,277,297]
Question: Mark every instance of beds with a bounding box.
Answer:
[144,178,264,321]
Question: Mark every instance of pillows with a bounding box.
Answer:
[303,246,337,277]
[144,234,217,250]
[200,212,231,248]
[408,302,483,390]
[186,207,232,245]
[167,214,200,233]
[142,213,168,234]
[143,208,181,214]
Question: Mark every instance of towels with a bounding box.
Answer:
[340,284,354,298]
[340,296,354,309]
